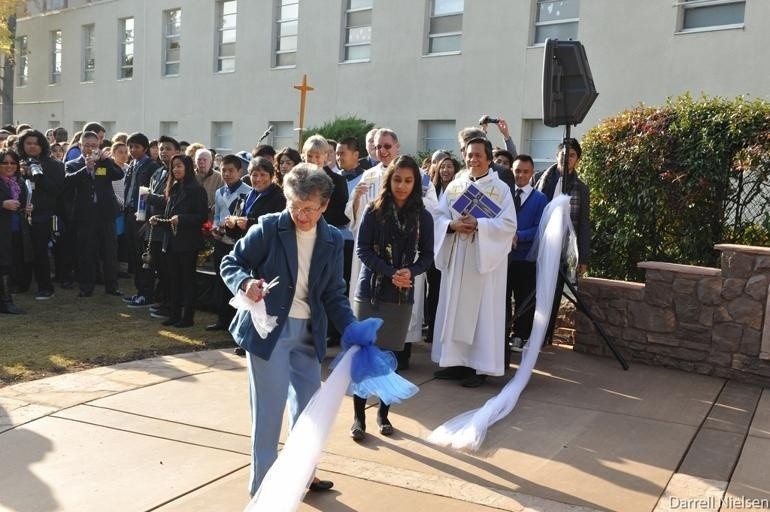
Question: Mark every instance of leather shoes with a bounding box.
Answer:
[234,347,246,356]
[350,422,367,439]
[462,372,486,388]
[77,288,95,296]
[208,320,228,331]
[61,279,74,288]
[434,366,476,380]
[310,478,335,490]
[106,287,124,297]
[120,272,132,280]
[377,421,393,435]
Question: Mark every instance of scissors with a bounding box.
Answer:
[254,276,280,303]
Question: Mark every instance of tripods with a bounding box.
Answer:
[507,126,631,372]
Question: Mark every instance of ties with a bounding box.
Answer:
[515,189,523,210]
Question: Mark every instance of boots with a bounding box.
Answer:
[1,275,28,314]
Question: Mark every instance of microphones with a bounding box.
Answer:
[258,125,277,140]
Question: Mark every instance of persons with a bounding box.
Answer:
[62,131,124,297]
[422,156,436,342]
[208,148,216,168]
[350,154,434,439]
[8,129,66,300]
[179,141,190,154]
[273,146,301,188]
[145,141,165,166]
[428,149,451,198]
[433,137,516,388]
[458,127,515,369]
[194,149,225,312]
[212,153,223,172]
[533,138,590,347]
[241,143,276,186]
[344,127,439,371]
[327,136,372,348]
[302,134,350,227]
[424,157,460,342]
[52,131,123,296]
[206,155,253,338]
[111,141,131,279]
[509,154,548,348]
[225,156,287,355]
[325,139,340,173]
[142,135,181,319]
[46,130,55,145]
[480,117,521,160]
[359,129,380,170]
[64,122,111,283]
[1,135,20,147]
[50,143,78,288]
[149,154,207,328]
[492,149,515,170]
[219,163,359,499]
[121,133,159,309]
[253,145,274,156]
[185,143,205,168]
[49,127,70,158]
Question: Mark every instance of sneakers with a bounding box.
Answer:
[9,283,30,294]
[512,335,524,351]
[150,305,162,311]
[161,314,183,326]
[127,295,153,309]
[174,316,196,329]
[122,296,134,303]
[150,307,182,318]
[36,288,56,300]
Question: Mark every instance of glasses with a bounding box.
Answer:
[1,161,18,165]
[284,202,327,214]
[376,143,398,151]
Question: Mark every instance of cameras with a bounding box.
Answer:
[90,149,100,158]
[27,156,45,178]
[479,113,500,126]
